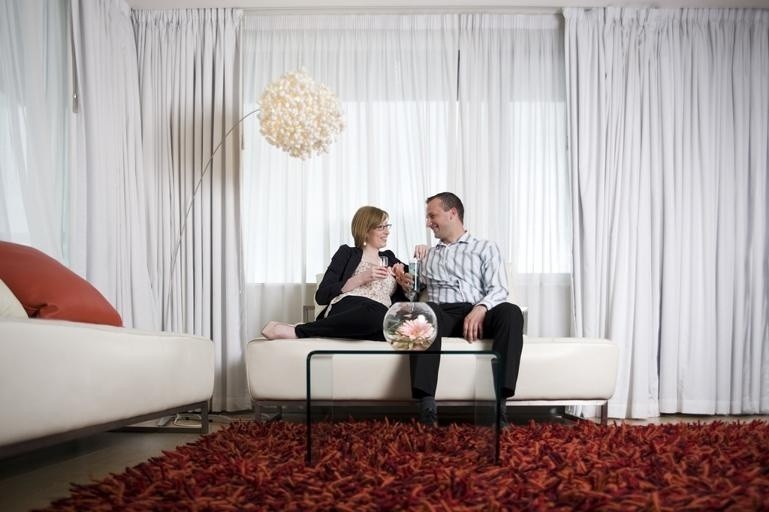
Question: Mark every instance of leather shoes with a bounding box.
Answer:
[261,321,306,340]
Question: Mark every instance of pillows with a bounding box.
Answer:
[0,240,125,327]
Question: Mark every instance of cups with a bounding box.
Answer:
[408,260,422,294]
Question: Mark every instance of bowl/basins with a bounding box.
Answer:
[377,256,388,279]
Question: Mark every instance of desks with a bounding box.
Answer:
[303,347,503,469]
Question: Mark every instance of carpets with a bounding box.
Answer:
[42,410,769,510]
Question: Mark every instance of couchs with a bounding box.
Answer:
[0,237,219,464]
[247,269,616,429]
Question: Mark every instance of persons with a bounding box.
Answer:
[401,191,524,429]
[261,205,428,342]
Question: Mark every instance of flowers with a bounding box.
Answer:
[387,314,435,351]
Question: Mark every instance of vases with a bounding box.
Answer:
[382,301,439,351]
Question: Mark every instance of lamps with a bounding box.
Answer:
[151,65,352,337]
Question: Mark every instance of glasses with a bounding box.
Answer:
[377,224,391,230]
